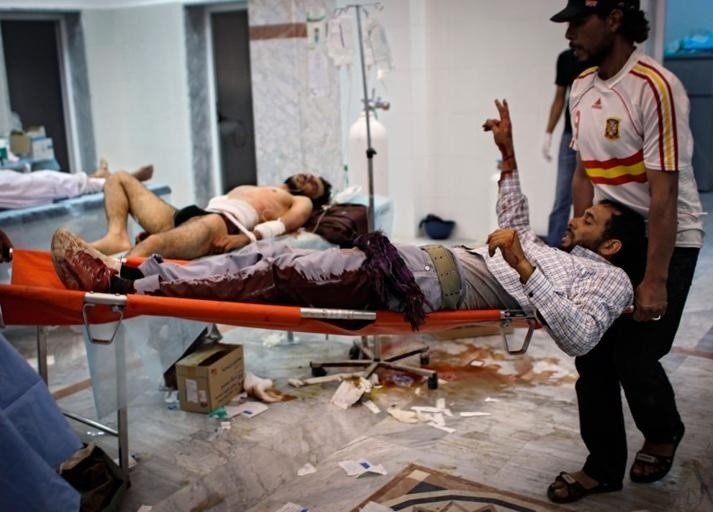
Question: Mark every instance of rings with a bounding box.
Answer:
[651,314,663,322]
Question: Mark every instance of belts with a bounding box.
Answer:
[419,243,464,313]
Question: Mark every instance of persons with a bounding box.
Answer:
[548,1,709,505]
[50,99,645,355]
[543,50,601,248]
[0,158,154,210]
[86,170,335,257]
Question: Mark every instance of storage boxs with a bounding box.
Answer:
[11,125,45,155]
[175,343,244,414]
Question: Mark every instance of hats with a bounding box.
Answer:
[549,0,640,23]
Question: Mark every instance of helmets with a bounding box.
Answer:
[425,212,455,239]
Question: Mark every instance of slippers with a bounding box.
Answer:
[548,471,624,505]
[626,418,686,483]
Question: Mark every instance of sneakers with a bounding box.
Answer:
[49,227,120,293]
[59,225,124,278]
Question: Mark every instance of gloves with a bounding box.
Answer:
[540,132,553,163]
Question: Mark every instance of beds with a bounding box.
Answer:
[1,183,172,284]
[37,193,391,489]
